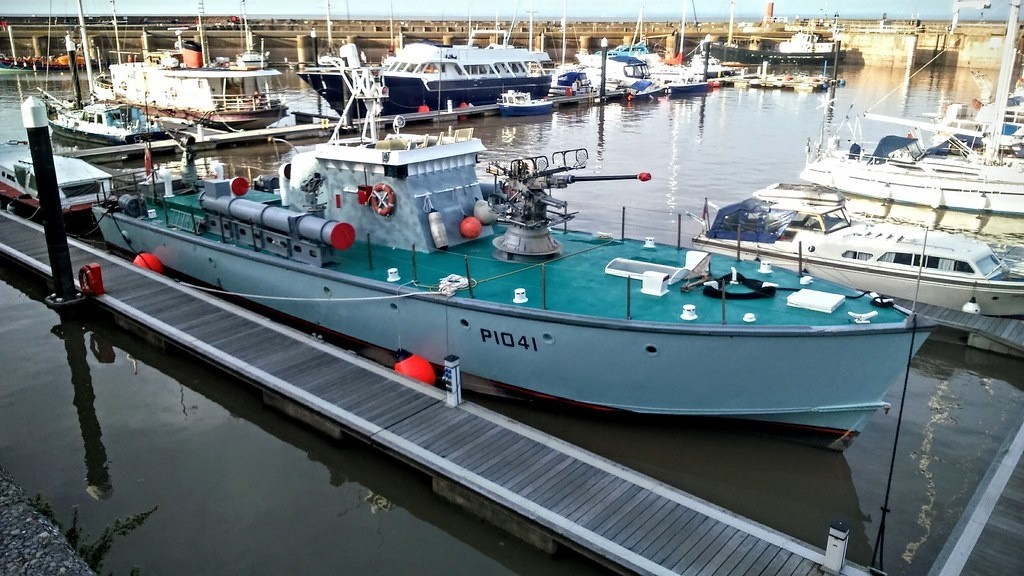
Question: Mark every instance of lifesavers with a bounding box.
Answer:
[79,267,95,294]
[370,182,397,217]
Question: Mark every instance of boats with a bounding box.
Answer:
[40,29,843,151]
[692,92,1024,313]
[95,130,921,447]
[2,152,121,229]
